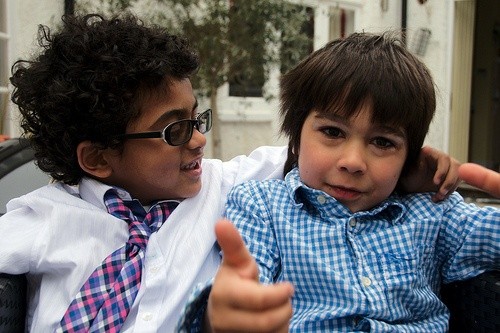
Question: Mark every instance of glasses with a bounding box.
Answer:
[92,109,212,147]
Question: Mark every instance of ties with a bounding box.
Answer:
[53,190,179,332]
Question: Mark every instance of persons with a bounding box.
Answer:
[1,10,462,333]
[169,30,500,333]
